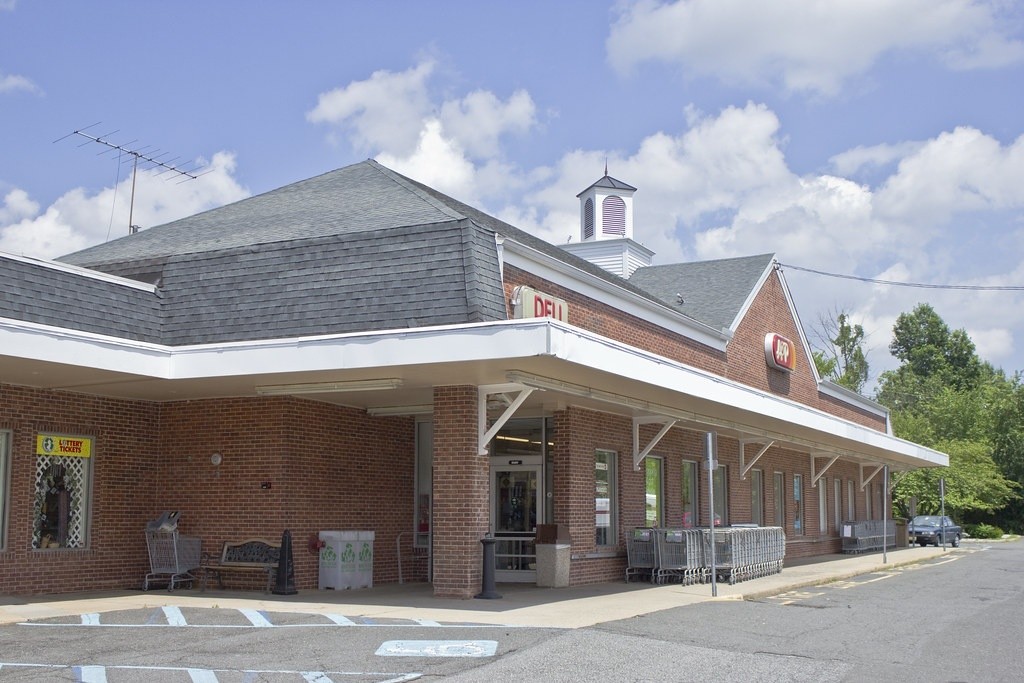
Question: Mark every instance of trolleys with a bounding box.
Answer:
[622,524,787,585]
[841,518,898,554]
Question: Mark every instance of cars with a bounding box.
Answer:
[907,515,963,547]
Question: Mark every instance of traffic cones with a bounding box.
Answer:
[271,529,300,595]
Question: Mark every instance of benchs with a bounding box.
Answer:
[199,538,282,595]
